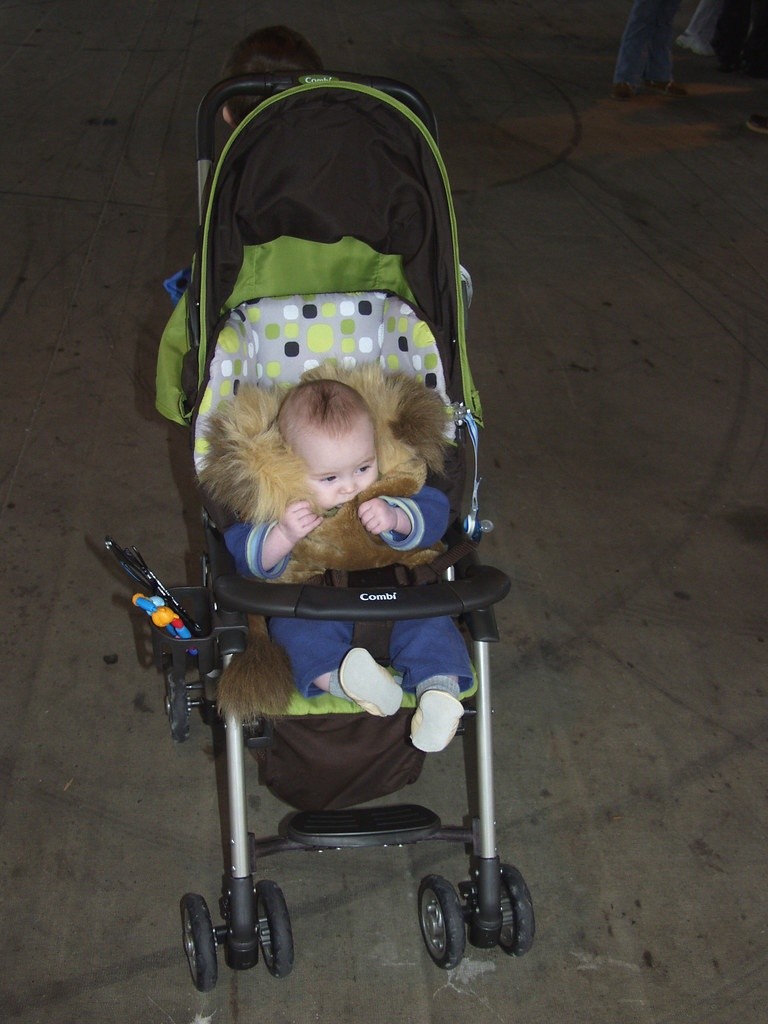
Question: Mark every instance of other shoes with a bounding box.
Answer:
[411,689,465,752]
[340,647,403,717]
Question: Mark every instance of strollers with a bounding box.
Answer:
[100,69,538,990]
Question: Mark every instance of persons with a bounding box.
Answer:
[609,0,689,97]
[197,357,477,753]
[676,0,768,134]
